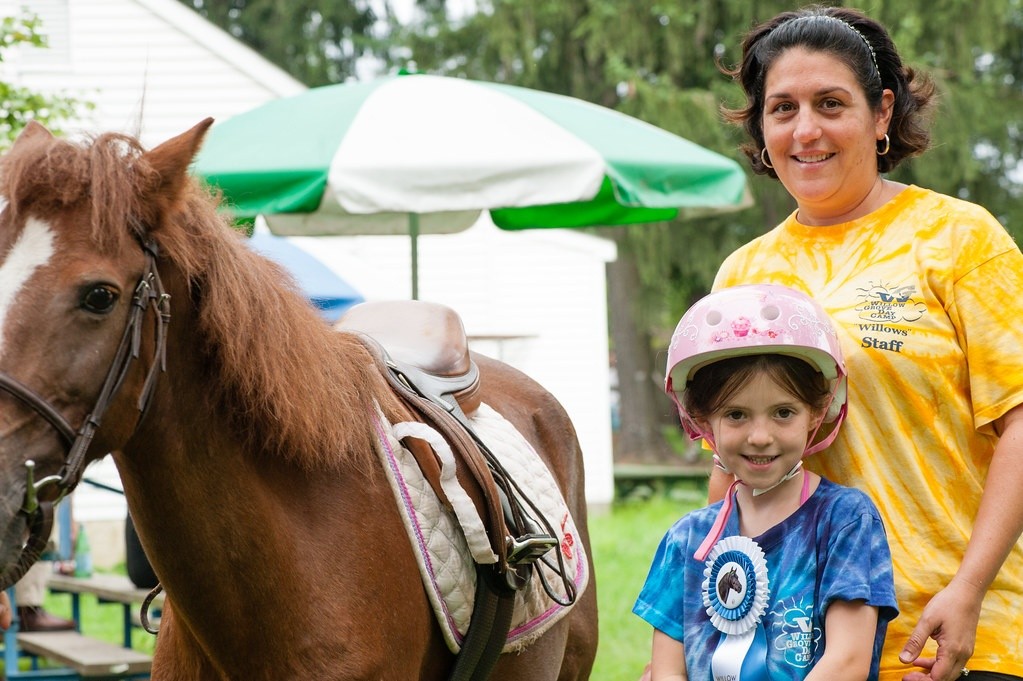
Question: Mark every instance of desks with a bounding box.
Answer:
[51,572,166,650]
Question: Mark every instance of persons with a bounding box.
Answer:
[0,590,13,630]
[14,529,76,632]
[709,3,1023,681]
[631,283,901,681]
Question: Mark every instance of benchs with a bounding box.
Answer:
[18,631,153,681]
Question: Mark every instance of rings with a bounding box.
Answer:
[960,667,969,676]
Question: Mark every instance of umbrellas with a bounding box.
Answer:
[184,67,755,301]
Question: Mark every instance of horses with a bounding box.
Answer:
[0,117,598,681]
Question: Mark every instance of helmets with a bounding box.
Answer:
[664,285,846,427]
